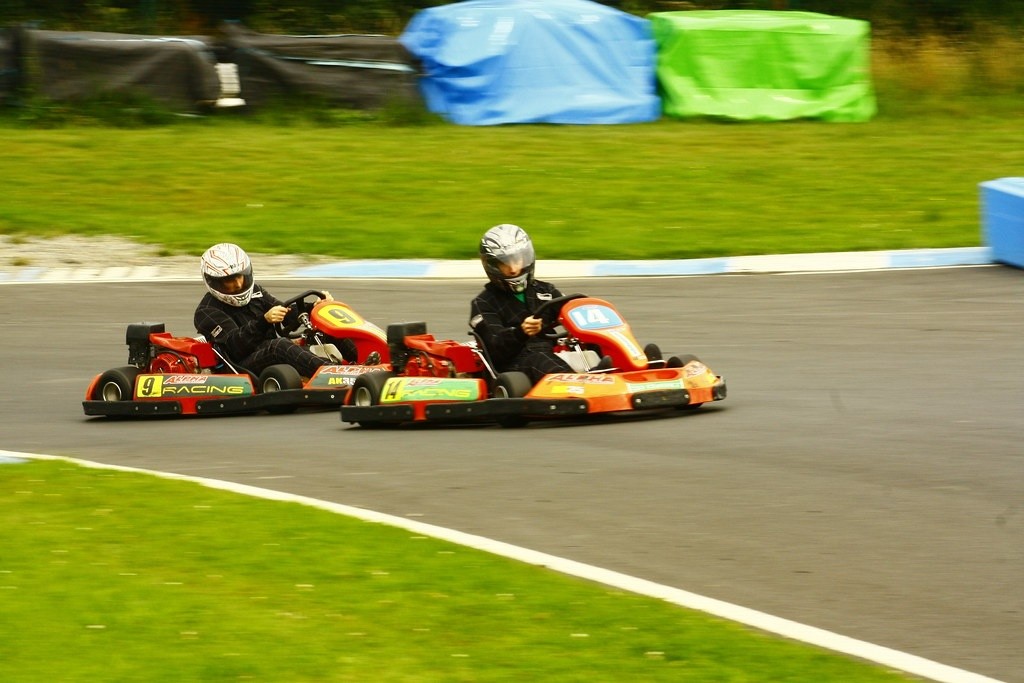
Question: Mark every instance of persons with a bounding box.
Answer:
[469,224,664,382]
[194,243,382,378]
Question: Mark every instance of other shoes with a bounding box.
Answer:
[587,356,613,374]
[644,344,666,369]
[362,350,381,366]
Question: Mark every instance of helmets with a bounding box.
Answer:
[479,224,535,294]
[200,242,255,307]
